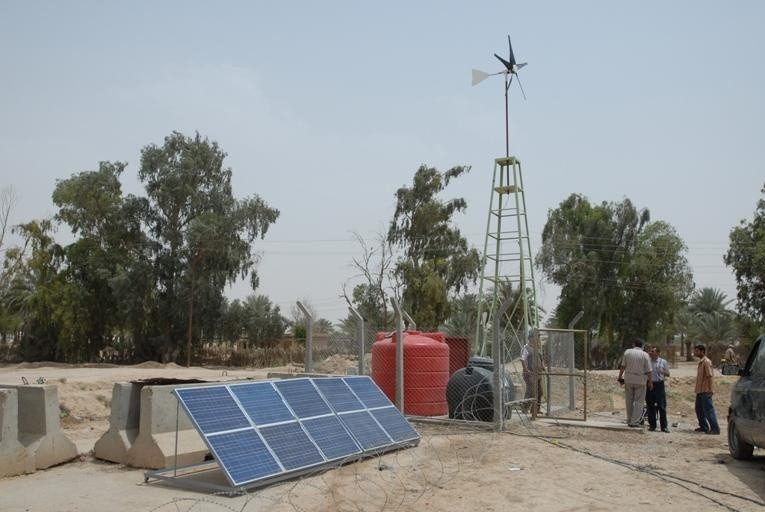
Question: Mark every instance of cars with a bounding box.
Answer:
[721,331,764,463]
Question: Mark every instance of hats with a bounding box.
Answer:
[529,328,540,336]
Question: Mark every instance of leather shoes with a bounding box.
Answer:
[661,428,669,432]
[695,428,709,431]
[705,430,720,434]
[649,428,655,431]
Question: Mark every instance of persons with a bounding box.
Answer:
[694,344,721,434]
[724,345,736,363]
[645,346,671,433]
[519,328,549,415]
[617,339,653,427]
